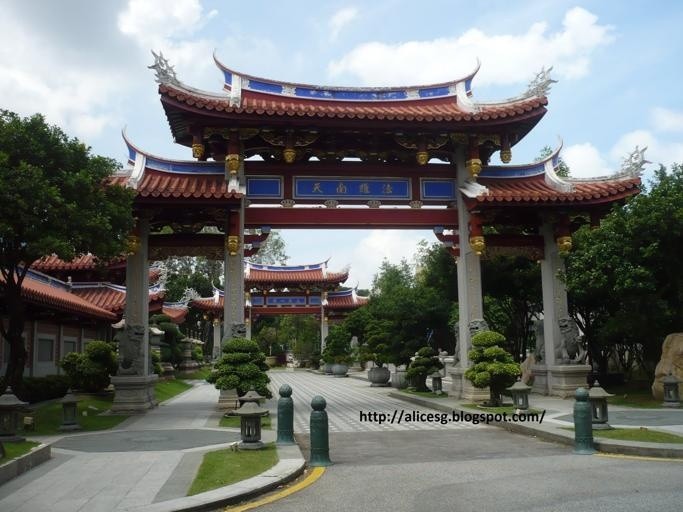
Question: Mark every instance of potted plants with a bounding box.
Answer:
[362,341,416,392]
[324,354,349,378]
